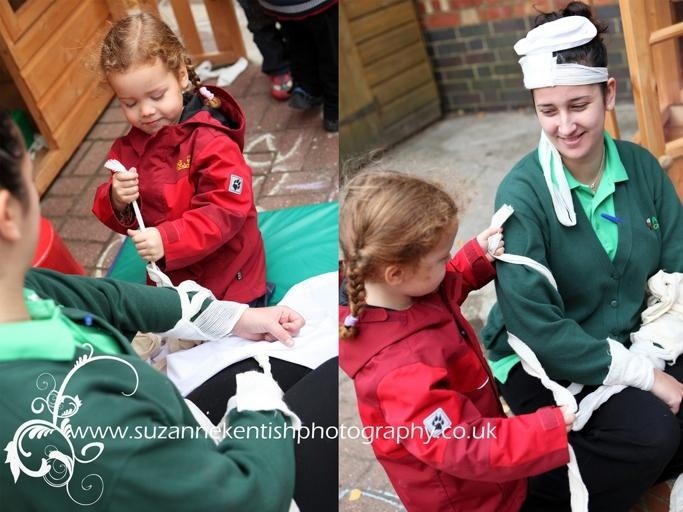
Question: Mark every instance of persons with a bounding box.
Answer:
[259,0,338,131]
[480,0,683,512]
[235,0,294,101]
[0,109,339,512]
[339,168,576,512]
[91,13,269,311]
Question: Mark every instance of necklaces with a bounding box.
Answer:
[591,146,606,188]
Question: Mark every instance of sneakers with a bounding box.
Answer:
[324,118,337,131]
[288,85,323,110]
[271,72,293,101]
